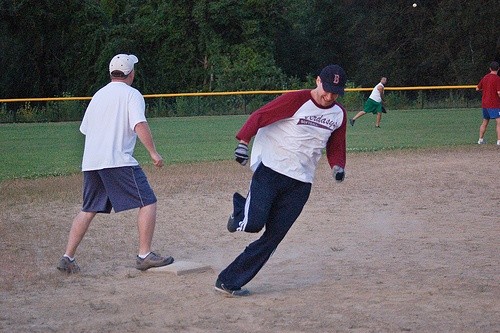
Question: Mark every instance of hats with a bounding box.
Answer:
[319,64,346,97]
[109,53,138,78]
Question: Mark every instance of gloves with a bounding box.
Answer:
[331,165,346,184]
[234,143,249,166]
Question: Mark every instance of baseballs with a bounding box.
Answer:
[413,3,417,8]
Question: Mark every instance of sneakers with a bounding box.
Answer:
[478,138,485,144]
[375,125,380,128]
[214,278,250,297]
[135,251,175,271]
[496,139,500,144]
[227,212,239,233]
[349,118,355,127]
[56,255,80,274]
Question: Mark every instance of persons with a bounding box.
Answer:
[56,53,175,273]
[476,59,500,146]
[215,64,347,297]
[349,76,387,129]
